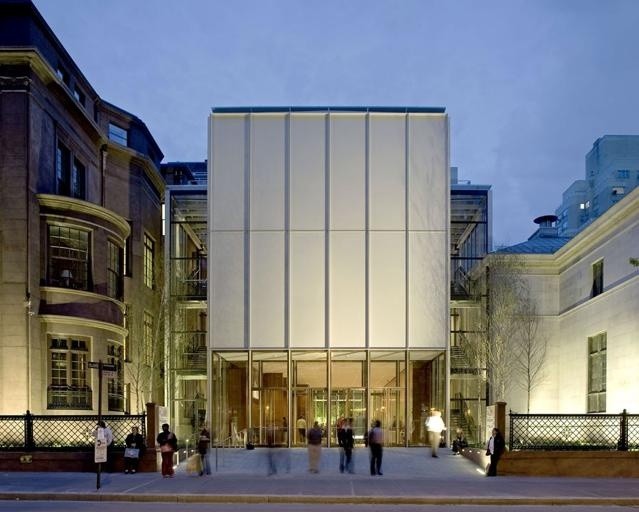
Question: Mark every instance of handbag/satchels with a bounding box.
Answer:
[124,446,139,458]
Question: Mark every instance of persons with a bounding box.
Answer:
[92,420,112,472]
[123,427,143,475]
[452,427,466,455]
[281,417,287,441]
[368,419,384,476]
[337,417,355,474]
[196,425,213,475]
[297,416,307,442]
[157,423,176,479]
[485,428,502,476]
[307,421,322,475]
[425,411,445,459]
